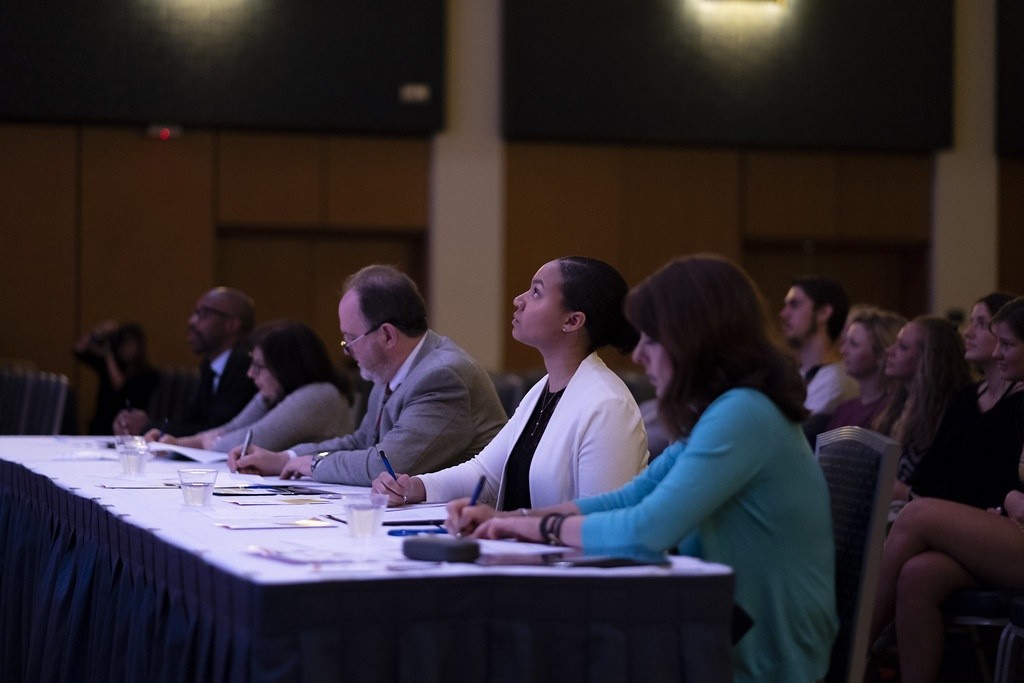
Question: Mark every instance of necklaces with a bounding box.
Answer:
[531,374,571,436]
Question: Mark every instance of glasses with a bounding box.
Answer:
[190,306,228,319]
[247,361,267,374]
[341,323,381,355]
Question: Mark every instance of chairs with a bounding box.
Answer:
[815,426,902,683]
[490,371,523,419]
[1,370,69,436]
[955,593,1024,683]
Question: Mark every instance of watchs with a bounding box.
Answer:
[311,452,329,471]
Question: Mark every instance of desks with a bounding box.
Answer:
[0,435,735,683]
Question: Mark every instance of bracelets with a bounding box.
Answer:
[540,512,574,545]
[518,508,527,516]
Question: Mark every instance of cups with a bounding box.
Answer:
[177,469,218,507]
[343,492,390,545]
[115,436,149,475]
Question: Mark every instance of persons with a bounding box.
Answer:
[227,265,509,486]
[73,318,169,436]
[444,255,837,683]
[113,287,259,437]
[371,258,651,511]
[146,318,356,453]
[781,280,1024,683]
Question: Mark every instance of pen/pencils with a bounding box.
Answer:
[379,450,408,503]
[236,428,253,473]
[388,528,449,536]
[159,418,169,438]
[457,475,486,538]
